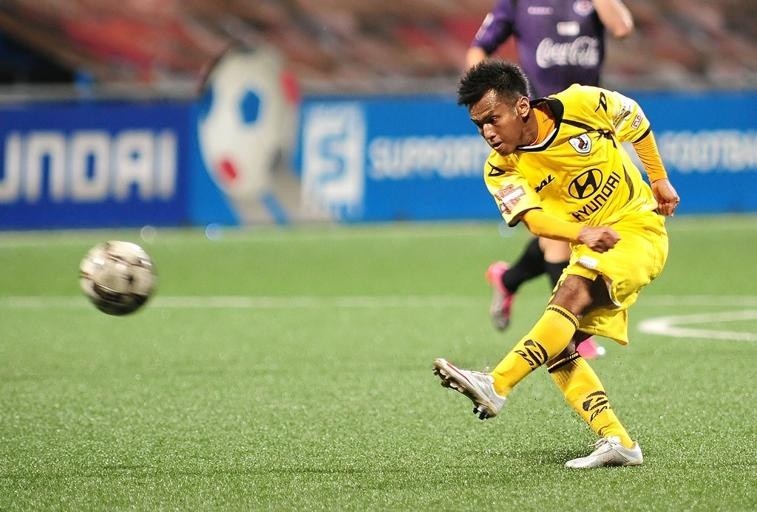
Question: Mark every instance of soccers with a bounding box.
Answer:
[80,241,155,317]
[196,44,300,201]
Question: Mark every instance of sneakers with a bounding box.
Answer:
[578,337,603,359]
[486,262,518,333]
[434,357,507,421]
[565,436,644,470]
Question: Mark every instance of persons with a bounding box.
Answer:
[463,0,635,362]
[433,56,680,468]
[0,0,757,95]
